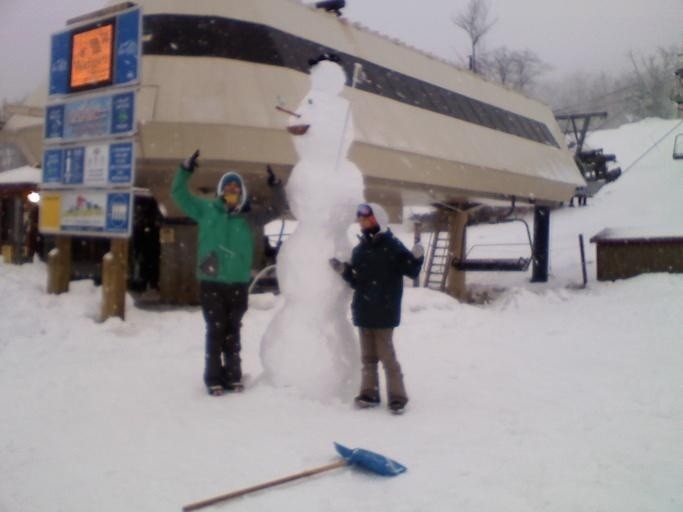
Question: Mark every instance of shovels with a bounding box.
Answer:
[182,442,406,511]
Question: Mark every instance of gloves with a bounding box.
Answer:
[263,163,282,185]
[411,243,425,260]
[183,147,201,170]
[330,258,347,275]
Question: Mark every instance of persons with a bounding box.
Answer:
[170,149,285,395]
[328,202,423,415]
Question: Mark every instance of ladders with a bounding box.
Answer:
[423,208,454,293]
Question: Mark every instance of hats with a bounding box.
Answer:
[355,203,374,217]
[223,175,241,188]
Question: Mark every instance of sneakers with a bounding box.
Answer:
[205,378,226,397]
[356,392,380,407]
[386,395,408,415]
[225,378,244,393]
